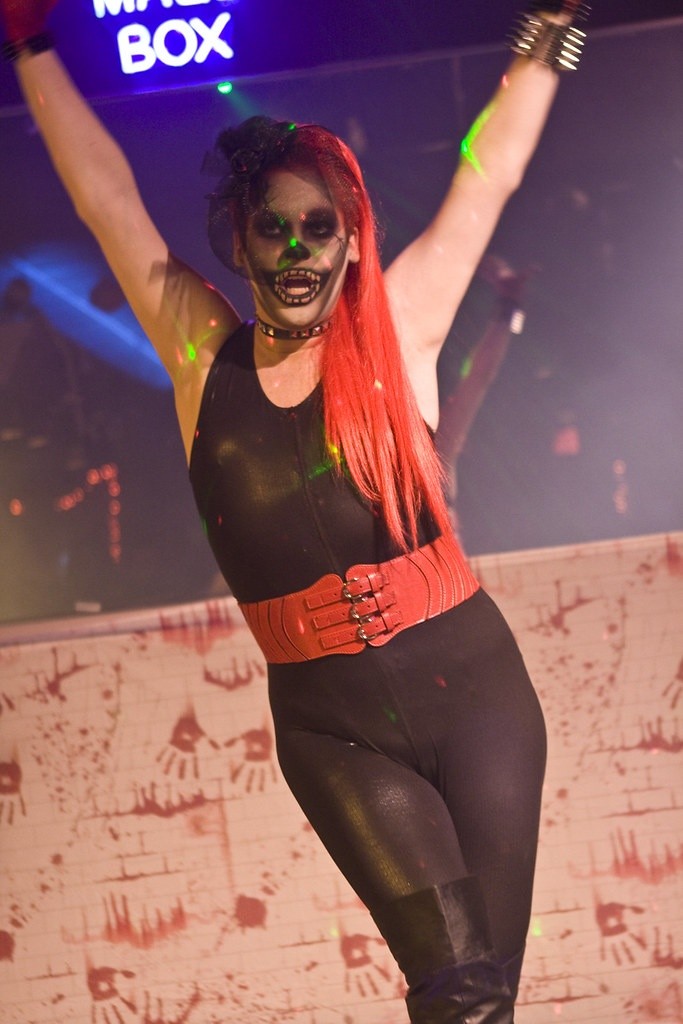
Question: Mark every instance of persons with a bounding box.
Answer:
[0,1,597,1024]
[418,257,526,555]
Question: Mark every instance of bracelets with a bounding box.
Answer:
[492,277,520,320]
[513,15,586,72]
[8,31,57,60]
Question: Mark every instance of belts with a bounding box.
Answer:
[239,531,482,663]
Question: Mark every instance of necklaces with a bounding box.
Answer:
[255,314,332,340]
